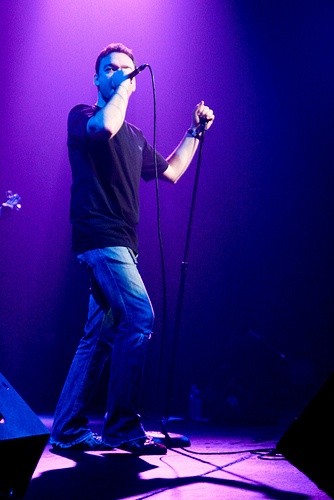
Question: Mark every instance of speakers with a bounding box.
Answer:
[0,371,52,500]
[276,372,334,499]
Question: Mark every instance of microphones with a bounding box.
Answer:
[128,64,147,80]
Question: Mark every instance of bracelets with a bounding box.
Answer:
[187,128,201,139]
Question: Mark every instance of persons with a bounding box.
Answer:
[49,43,215,454]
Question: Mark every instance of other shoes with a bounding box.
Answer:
[52,436,114,451]
[119,436,167,454]
